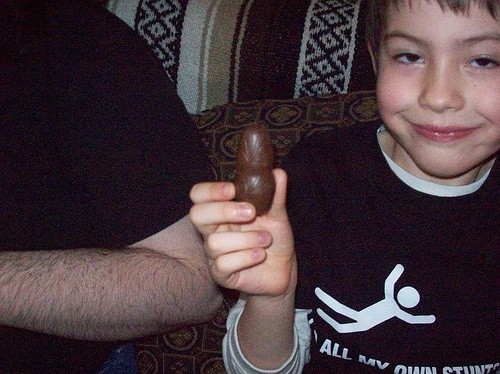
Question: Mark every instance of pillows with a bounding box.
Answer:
[134,90,380,374]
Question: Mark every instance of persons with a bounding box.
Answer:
[0,0,220,374]
[190,0,500,374]
